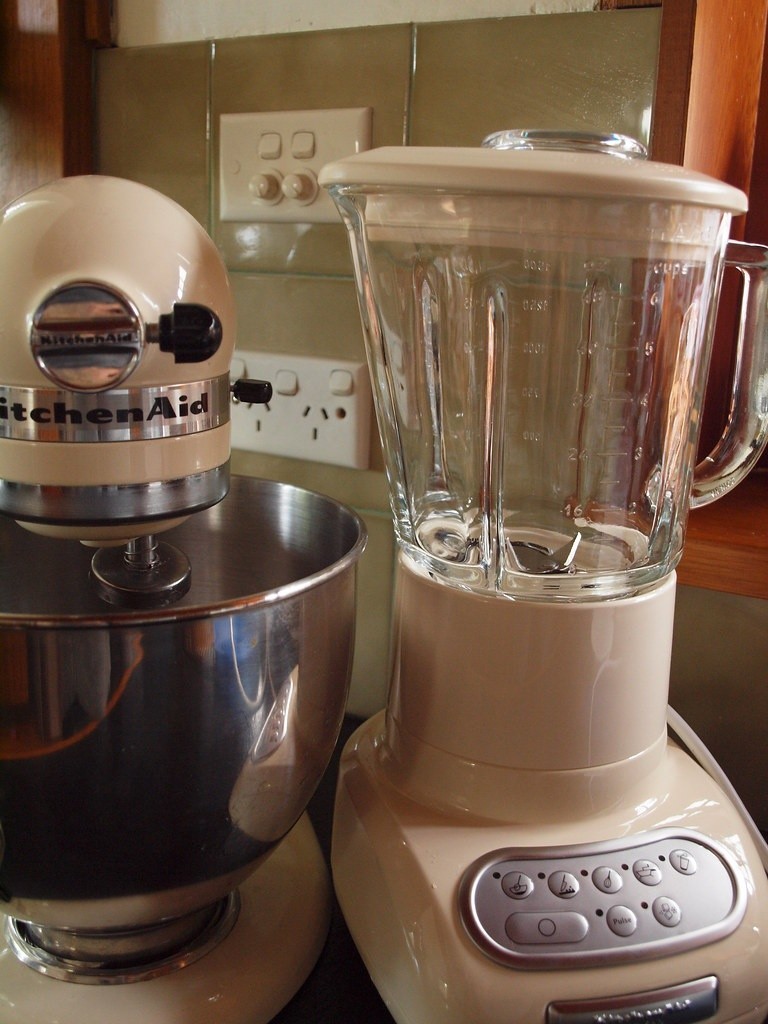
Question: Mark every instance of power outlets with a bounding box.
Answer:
[230,352,372,472]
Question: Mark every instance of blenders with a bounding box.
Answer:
[318,124,768,1024]
